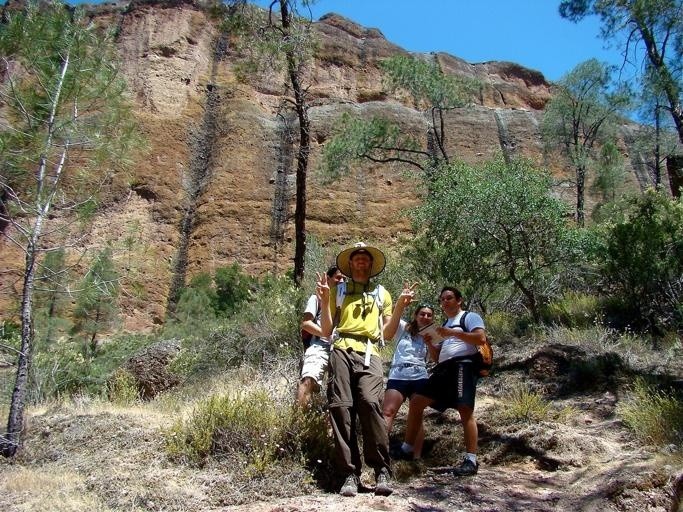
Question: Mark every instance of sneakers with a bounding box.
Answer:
[373,470,393,495]
[339,472,358,496]
[451,458,478,477]
[389,446,416,462]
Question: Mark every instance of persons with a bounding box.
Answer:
[382,305,439,459]
[314,240,421,496]
[296,265,347,413]
[400,286,487,475]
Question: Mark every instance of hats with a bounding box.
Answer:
[334,241,386,278]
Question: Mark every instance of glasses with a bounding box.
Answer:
[418,303,434,309]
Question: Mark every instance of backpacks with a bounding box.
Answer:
[438,310,494,377]
[300,293,322,344]
